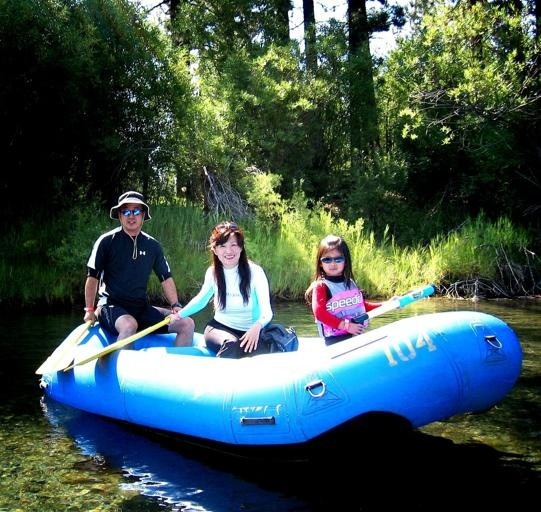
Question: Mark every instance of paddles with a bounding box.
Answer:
[34,304,102,374]
[64,317,171,373]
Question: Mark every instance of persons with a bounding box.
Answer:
[84,191,195,350]
[166,223,273,359]
[305,236,402,347]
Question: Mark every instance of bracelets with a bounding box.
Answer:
[343,319,349,331]
[172,303,182,308]
[85,308,94,311]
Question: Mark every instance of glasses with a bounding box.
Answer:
[215,222,238,231]
[321,256,345,263]
[119,209,145,217]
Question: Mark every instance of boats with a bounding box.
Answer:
[38,312,522,448]
[40,395,303,511]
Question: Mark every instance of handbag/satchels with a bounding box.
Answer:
[264,323,299,351]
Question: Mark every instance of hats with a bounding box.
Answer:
[110,191,152,221]
